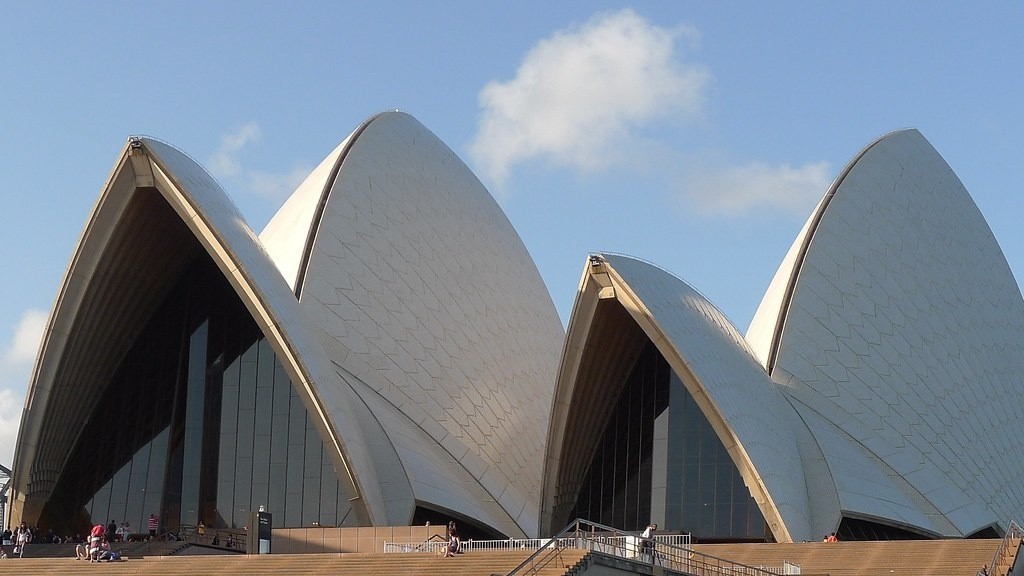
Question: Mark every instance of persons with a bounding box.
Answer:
[0,513,236,563]
[823,532,838,543]
[980,564,988,576]
[443,521,460,557]
[639,524,657,556]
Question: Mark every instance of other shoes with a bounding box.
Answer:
[96,560,101,563]
[76,557,80,560]
[85,557,90,560]
[89,560,93,563]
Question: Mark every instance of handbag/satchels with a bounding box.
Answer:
[25,528,29,543]
[13,546,20,555]
